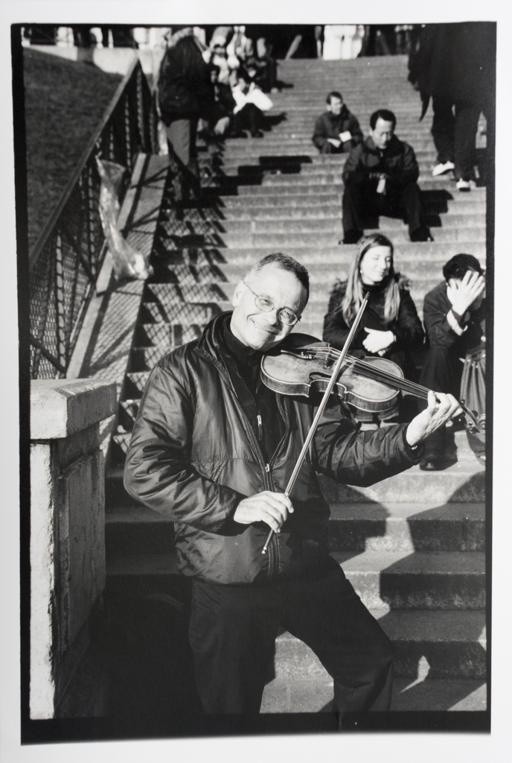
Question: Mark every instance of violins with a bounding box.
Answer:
[260,333,485,433]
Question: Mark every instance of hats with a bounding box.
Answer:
[443,254,484,279]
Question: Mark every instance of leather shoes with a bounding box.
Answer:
[420,452,457,471]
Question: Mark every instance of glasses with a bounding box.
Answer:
[242,281,300,327]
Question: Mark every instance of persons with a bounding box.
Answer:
[419,253,491,471]
[338,110,434,244]
[132,27,278,209]
[406,23,494,191]
[312,92,363,153]
[322,232,424,423]
[366,25,421,57]
[123,252,463,733]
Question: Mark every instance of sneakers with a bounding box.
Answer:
[432,161,455,176]
[456,178,471,191]
